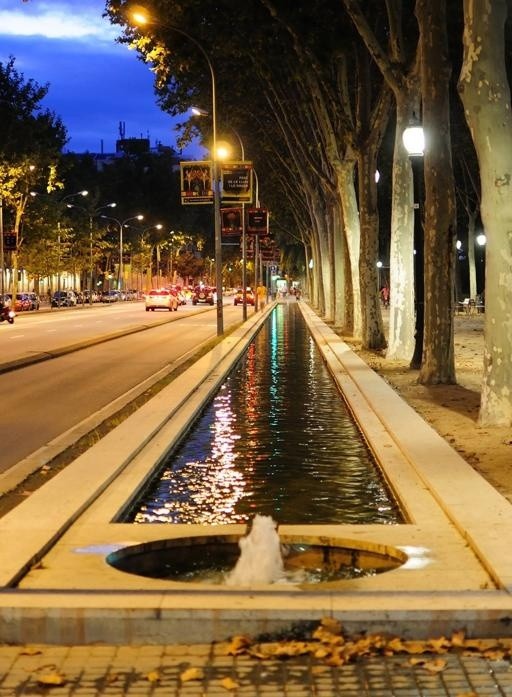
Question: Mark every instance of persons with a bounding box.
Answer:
[379,283,390,310]
[256,280,269,314]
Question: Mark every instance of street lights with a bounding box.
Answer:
[401,111,425,370]
[100,215,163,289]
[215,140,259,311]
[192,106,247,321]
[30,190,88,308]
[126,6,223,335]
[66,203,117,305]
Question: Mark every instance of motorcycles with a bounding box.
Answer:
[380,294,389,310]
[0,305,15,324]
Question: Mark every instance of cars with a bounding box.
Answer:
[0,292,32,311]
[141,285,255,311]
[51,289,140,308]
